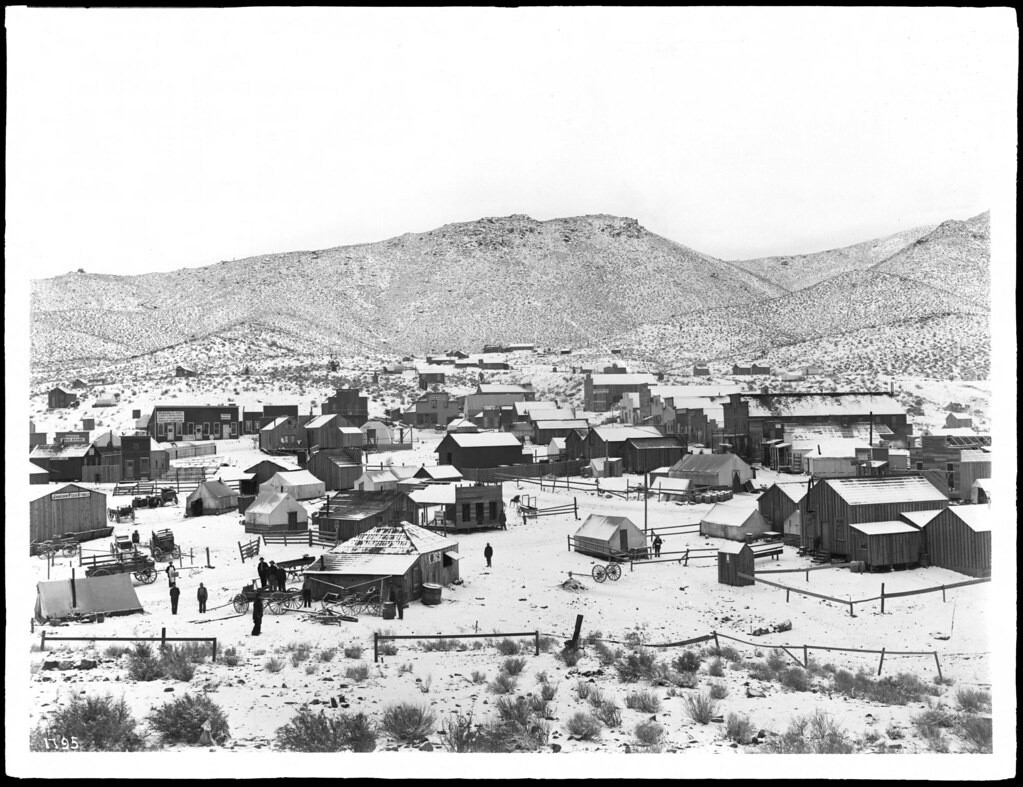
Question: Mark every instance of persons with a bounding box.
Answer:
[252,593,263,636]
[258,557,288,593]
[197,583,209,613]
[653,535,661,557]
[302,576,312,607]
[396,585,406,618]
[131,530,141,544]
[169,582,181,616]
[484,543,493,567]
[165,561,177,588]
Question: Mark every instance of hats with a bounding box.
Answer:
[269,560,275,564]
[169,561,173,564]
[259,557,264,560]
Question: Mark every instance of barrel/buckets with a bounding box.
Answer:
[383,601,395,619]
[745,533,754,543]
[695,490,733,504]
